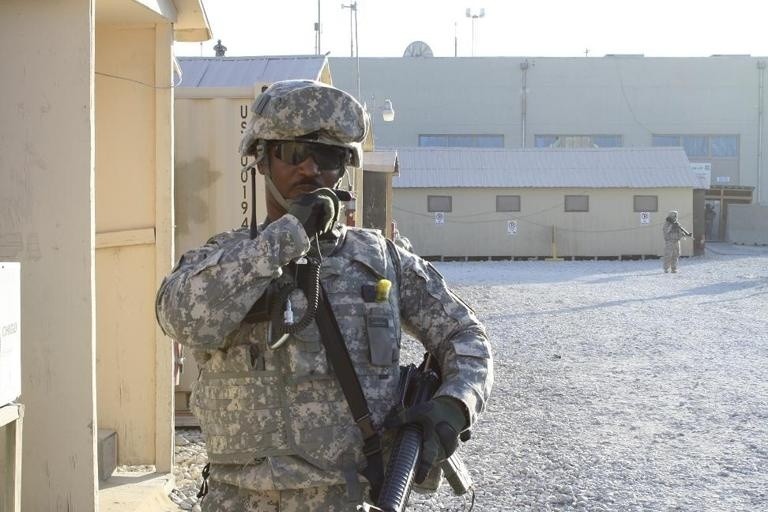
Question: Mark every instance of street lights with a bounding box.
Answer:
[466,7,487,56]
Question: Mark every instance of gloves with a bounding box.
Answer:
[385,395,472,486]
[289,185,352,244]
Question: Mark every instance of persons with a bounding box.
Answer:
[154,77,493,510]
[705,203,717,239]
[662,210,686,274]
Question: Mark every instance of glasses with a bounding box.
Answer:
[260,138,353,170]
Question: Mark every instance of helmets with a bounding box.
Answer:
[238,78,372,170]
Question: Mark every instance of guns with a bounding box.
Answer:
[377,353,472,512]
[666,217,692,238]
